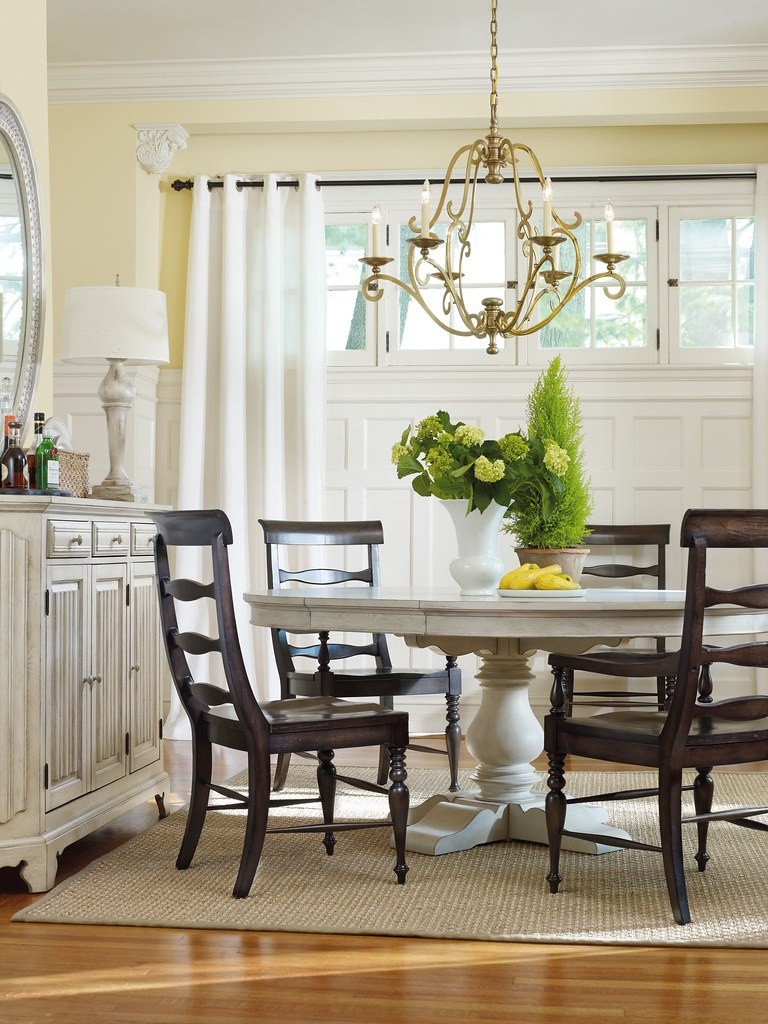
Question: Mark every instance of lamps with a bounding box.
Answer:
[59,274,171,502]
[358,0,629,356]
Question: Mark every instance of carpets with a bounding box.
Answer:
[12,758,768,950]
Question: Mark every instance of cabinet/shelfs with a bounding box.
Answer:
[1,495,174,893]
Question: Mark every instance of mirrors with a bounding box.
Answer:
[0,93,43,456]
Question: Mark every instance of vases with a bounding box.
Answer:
[439,499,515,597]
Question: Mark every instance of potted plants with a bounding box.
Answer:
[501,353,595,585]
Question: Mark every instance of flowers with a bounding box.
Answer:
[390,410,571,526]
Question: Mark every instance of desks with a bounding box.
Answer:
[242,587,768,853]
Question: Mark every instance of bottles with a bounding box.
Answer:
[26,413,45,489]
[35,429,59,489]
[0,415,29,488]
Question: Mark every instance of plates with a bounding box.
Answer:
[496,588,589,598]
[44,417,72,451]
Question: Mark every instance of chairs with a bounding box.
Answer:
[543,508,768,925]
[258,519,463,793]
[144,510,410,899]
